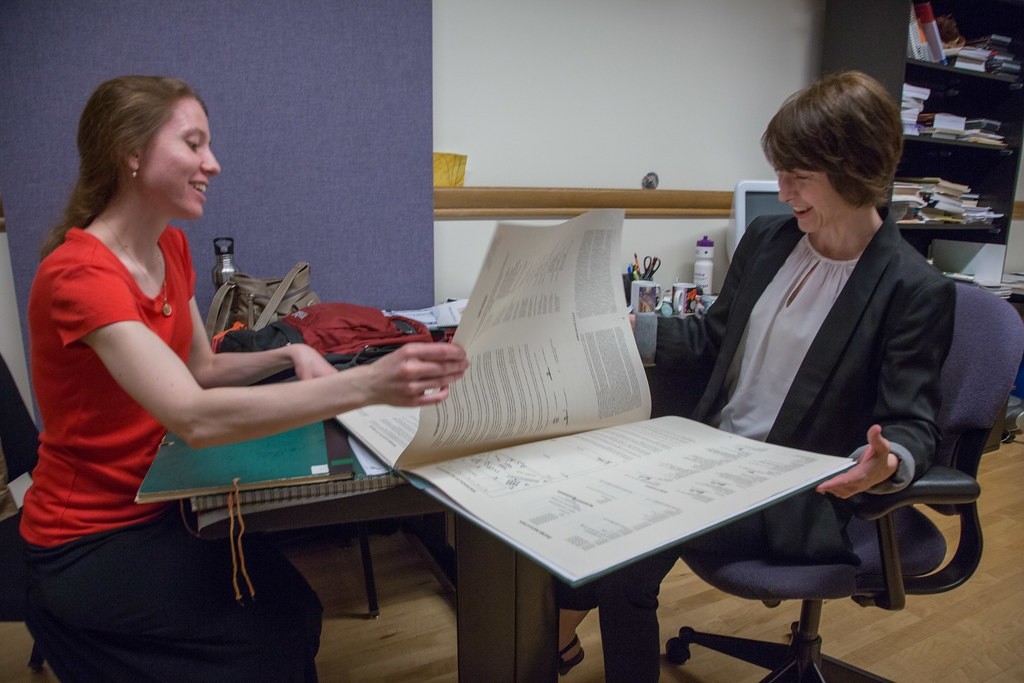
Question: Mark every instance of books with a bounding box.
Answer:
[900,81,966,140]
[134,418,408,512]
[891,176,979,223]
[335,208,860,588]
[906,2,1021,72]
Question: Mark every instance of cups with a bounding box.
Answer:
[631,280,664,312]
[673,283,697,317]
[695,295,718,319]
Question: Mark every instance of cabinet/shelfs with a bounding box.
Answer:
[823,0,1024,294]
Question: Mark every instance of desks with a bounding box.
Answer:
[197,419,560,683]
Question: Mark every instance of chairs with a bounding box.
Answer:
[0,353,47,675]
[665,281,1024,683]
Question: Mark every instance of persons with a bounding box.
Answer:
[18,76,470,683]
[556,71,958,683]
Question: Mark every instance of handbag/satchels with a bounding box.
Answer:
[205,260,320,344]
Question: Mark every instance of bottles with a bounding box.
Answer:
[211,238,240,290]
[693,236,716,295]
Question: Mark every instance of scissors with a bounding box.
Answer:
[644,256,661,281]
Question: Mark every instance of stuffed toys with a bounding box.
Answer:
[936,16,966,48]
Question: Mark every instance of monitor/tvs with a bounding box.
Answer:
[726,180,794,265]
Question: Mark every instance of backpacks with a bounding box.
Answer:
[217,300,457,386]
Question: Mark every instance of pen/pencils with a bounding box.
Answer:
[644,257,658,280]
[634,253,638,264]
[632,271,639,280]
[628,266,632,282]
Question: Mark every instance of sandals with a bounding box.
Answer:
[559,635,586,677]
